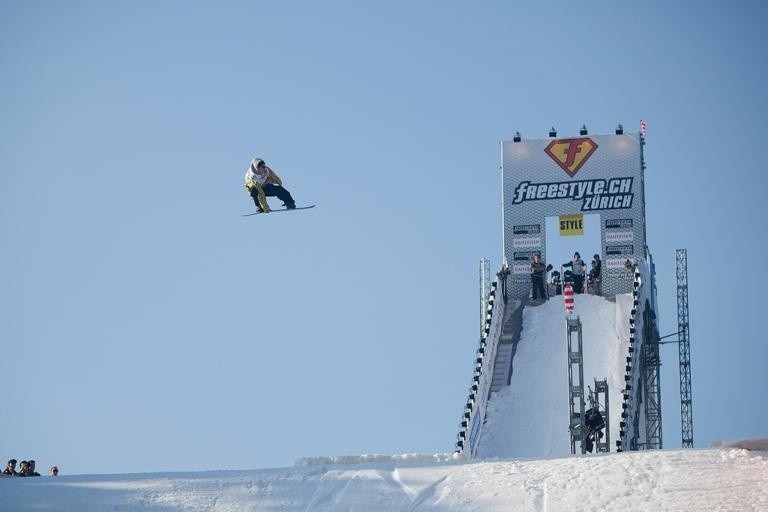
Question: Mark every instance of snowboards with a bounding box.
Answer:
[244,206,314,216]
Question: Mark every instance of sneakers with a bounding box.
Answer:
[286,203,296,208]
[256,207,263,212]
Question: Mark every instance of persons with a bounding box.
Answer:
[2,459,58,478]
[529,252,601,301]
[245,157,296,213]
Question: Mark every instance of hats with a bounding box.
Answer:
[253,159,264,170]
